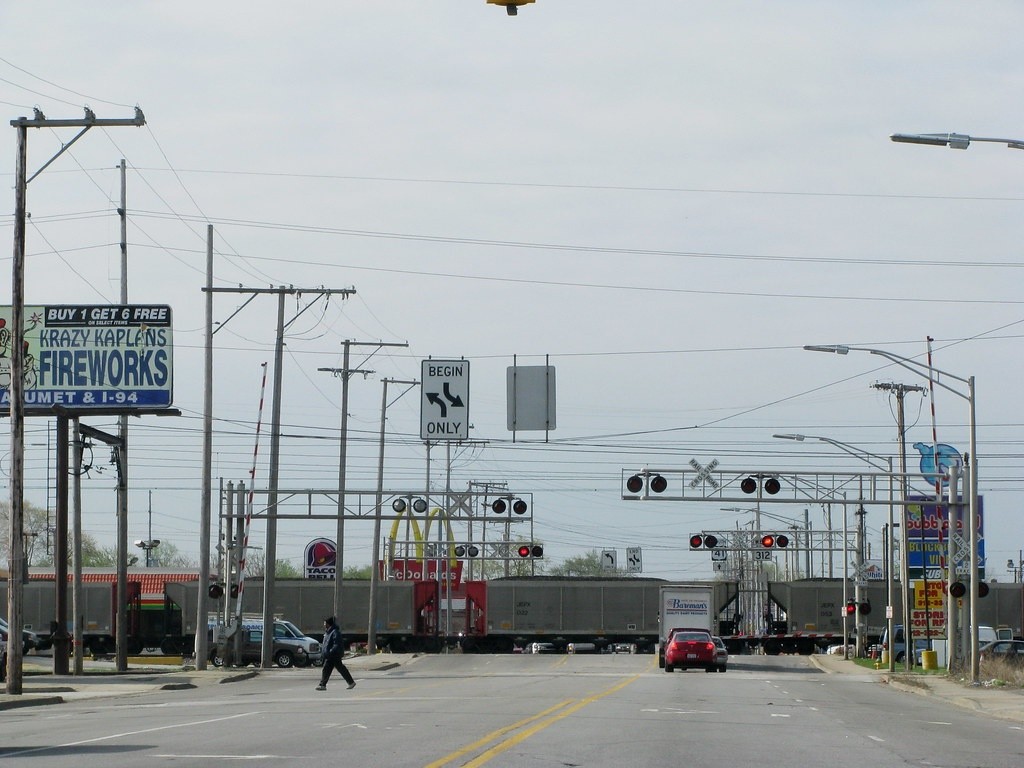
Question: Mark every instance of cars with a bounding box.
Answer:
[868,624,1024,667]
[659,628,727,673]
[210,628,306,669]
[0,618,40,680]
[616,644,631,654]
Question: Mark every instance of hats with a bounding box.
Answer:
[325,617,335,625]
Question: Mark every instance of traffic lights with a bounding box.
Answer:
[533,545,545,557]
[628,477,642,493]
[846,603,855,614]
[704,536,717,547]
[860,603,871,615]
[652,475,667,494]
[518,546,530,559]
[493,500,506,513]
[765,478,781,496]
[950,582,965,598]
[763,536,772,547]
[776,534,789,548]
[978,584,990,599]
[690,536,703,549]
[741,479,756,494]
[513,499,527,515]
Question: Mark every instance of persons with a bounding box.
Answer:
[314,616,357,691]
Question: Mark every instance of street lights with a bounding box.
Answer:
[719,507,813,578]
[803,344,982,682]
[773,431,896,672]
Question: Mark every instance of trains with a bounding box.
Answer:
[1,574,1024,656]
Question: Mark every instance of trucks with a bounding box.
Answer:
[207,618,321,658]
[658,583,715,644]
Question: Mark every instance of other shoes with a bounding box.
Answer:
[346,681,356,689]
[315,686,326,691]
